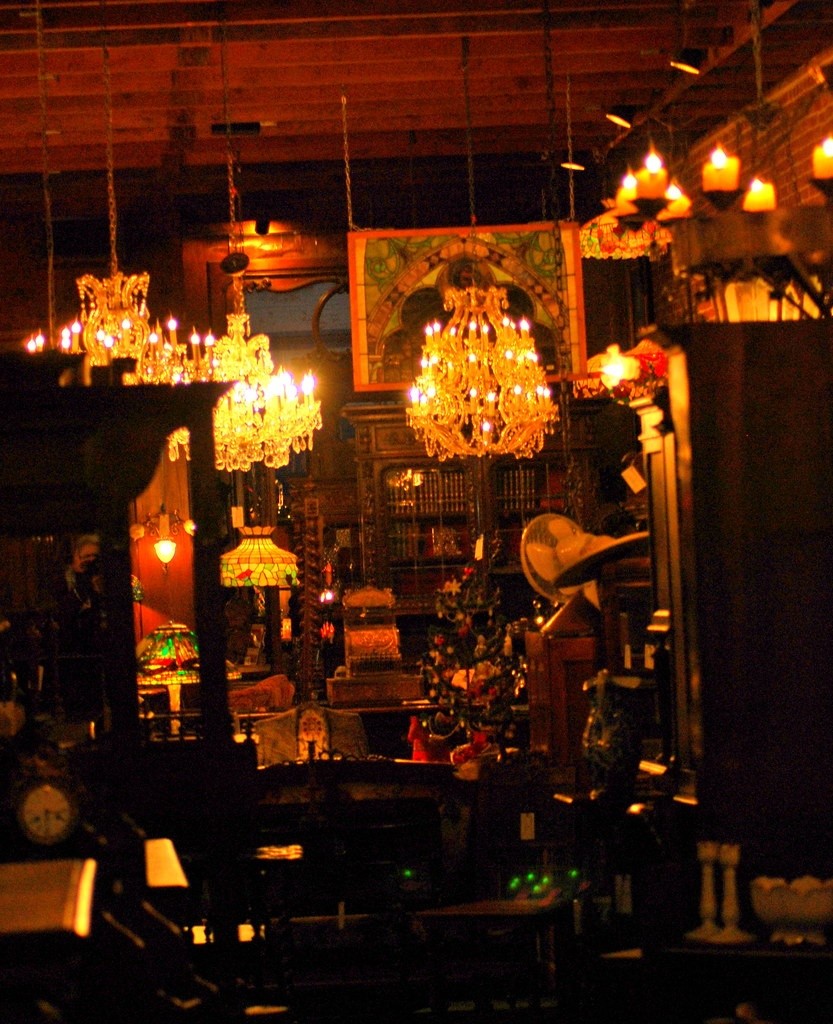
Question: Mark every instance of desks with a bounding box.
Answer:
[662,945,833,1023]
[230,704,484,718]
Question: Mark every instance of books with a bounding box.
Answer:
[390,516,532,555]
[386,469,539,514]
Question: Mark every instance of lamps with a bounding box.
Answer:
[219,463,302,587]
[129,503,196,575]
[572,339,669,400]
[405,33,560,460]
[135,621,202,743]
[611,0,833,231]
[580,198,674,261]
[22,0,323,474]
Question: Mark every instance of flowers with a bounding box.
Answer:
[417,530,533,770]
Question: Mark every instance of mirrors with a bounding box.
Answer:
[127,427,205,743]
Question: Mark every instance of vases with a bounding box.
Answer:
[747,876,833,945]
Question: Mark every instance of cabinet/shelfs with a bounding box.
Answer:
[524,631,601,787]
[628,322,833,881]
[0,384,233,742]
[342,401,604,618]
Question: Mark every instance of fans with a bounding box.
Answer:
[520,514,615,603]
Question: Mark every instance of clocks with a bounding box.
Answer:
[16,779,78,845]
